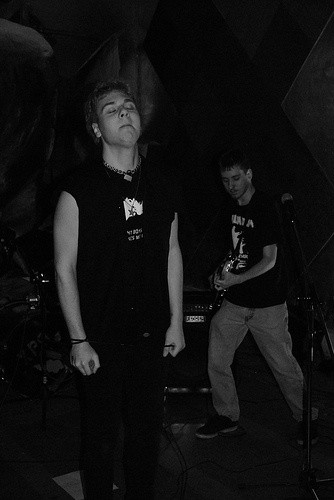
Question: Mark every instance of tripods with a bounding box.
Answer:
[238,220,334,500]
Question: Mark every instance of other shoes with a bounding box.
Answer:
[194,415,240,439]
[296,418,320,446]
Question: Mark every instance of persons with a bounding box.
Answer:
[196,151,318,445]
[54,81,186,500]
[0,225,36,279]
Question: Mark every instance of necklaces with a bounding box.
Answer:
[103,155,141,174]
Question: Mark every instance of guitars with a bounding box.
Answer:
[208,254,240,311]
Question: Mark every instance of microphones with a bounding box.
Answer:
[281,192,300,227]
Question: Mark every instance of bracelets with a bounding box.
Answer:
[71,337,89,344]
